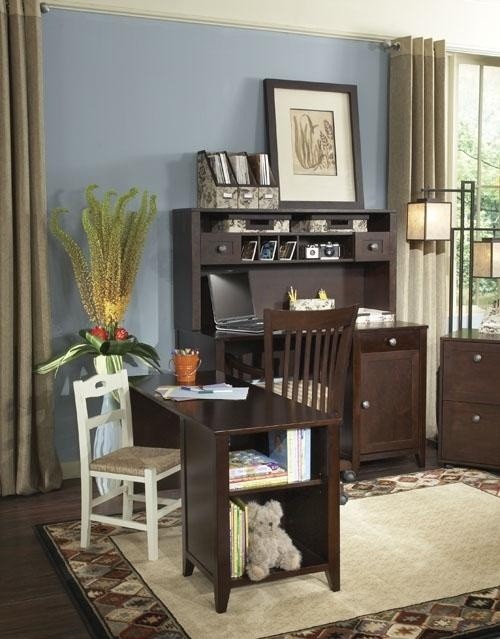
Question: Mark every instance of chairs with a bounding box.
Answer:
[72,369,183,563]
[264,304,359,416]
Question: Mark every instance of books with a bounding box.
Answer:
[228,495,250,578]
[228,448,287,493]
[207,152,272,186]
[267,427,312,484]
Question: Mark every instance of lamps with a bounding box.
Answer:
[406,179,499,331]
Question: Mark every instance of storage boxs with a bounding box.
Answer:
[195,150,280,208]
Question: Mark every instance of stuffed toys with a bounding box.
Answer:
[245,497,304,582]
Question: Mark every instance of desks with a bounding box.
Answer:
[129,370,345,613]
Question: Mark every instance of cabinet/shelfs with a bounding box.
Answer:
[171,207,429,471]
[437,328,500,469]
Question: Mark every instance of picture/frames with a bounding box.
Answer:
[262,79,366,207]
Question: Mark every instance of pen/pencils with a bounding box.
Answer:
[181,386,213,393]
[287,285,297,300]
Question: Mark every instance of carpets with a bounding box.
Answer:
[30,467,500,639]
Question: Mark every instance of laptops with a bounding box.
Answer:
[208,270,274,334]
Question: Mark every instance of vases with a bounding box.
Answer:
[92,382,123,498]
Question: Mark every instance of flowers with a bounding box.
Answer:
[32,184,163,404]
